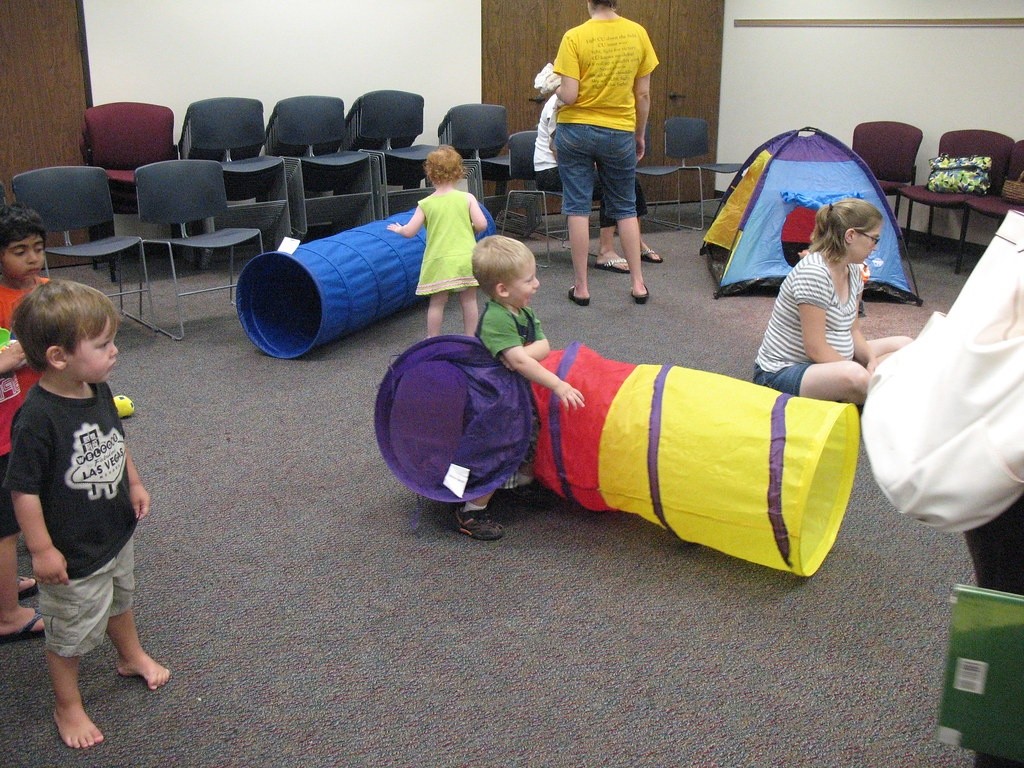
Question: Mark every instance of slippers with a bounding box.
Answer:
[17,578,38,600]
[641,249,663,263]
[595,259,631,274]
[0,608,46,644]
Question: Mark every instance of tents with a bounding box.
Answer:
[699,127,924,300]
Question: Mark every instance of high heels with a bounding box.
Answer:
[568,287,590,306]
[630,285,649,304]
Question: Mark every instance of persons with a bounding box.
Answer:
[451,235,585,541]
[752,198,914,412]
[0,203,52,644]
[553,0,659,307]
[533,93,663,273]
[387,144,487,339]
[0,279,171,749]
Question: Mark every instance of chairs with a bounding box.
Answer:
[898,129,1014,274]
[504,131,600,267]
[955,140,1024,273]
[663,116,744,230]
[9,88,546,341]
[853,121,924,251]
[634,122,704,233]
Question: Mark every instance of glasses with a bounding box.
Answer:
[854,229,880,244]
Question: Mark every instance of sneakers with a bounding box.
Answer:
[447,503,504,540]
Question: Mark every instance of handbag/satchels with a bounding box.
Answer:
[1001,171,1024,204]
[926,153,993,197]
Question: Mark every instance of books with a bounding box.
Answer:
[932,582,1024,763]
